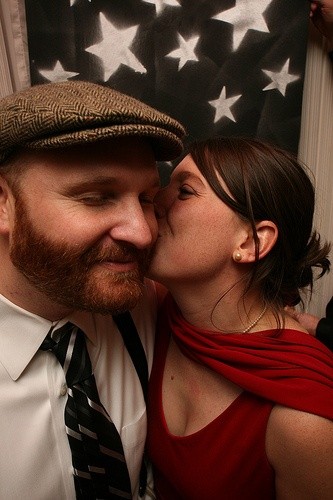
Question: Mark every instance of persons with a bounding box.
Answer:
[283,296,333,353]
[0,81,186,500]
[143,137,333,500]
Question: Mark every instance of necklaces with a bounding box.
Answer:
[242,295,268,333]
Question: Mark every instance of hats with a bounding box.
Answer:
[0,81,187,161]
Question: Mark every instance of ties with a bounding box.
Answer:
[40,322,131,500]
